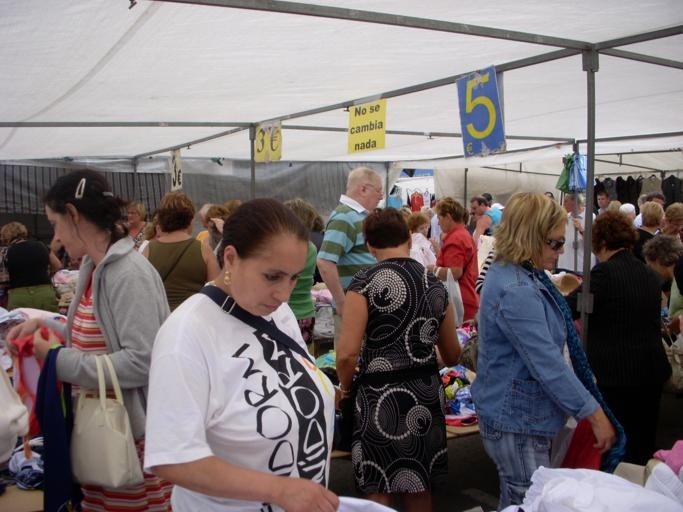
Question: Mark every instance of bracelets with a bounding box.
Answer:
[339,381,349,393]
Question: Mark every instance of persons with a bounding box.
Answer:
[309,214,325,284]
[638,231,683,398]
[467,190,628,512]
[332,206,463,512]
[140,196,341,511]
[1,223,79,314]
[314,166,384,320]
[470,191,504,244]
[195,200,242,254]
[145,192,221,311]
[126,203,155,252]
[286,200,320,346]
[561,194,596,274]
[429,198,480,324]
[583,209,666,465]
[597,191,683,239]
[403,206,444,239]
[3,167,177,511]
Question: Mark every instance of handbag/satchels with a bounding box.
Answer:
[339,381,349,393]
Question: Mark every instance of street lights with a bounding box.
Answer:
[68,352,147,491]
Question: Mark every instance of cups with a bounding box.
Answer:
[545,236,566,251]
